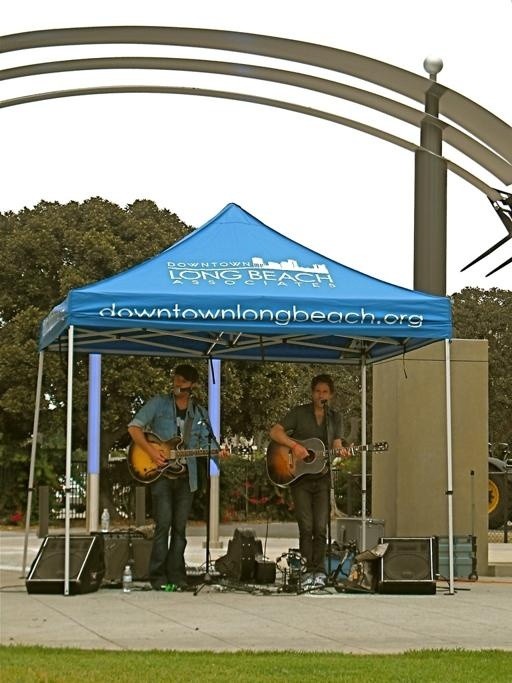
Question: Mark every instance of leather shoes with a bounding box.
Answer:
[150,579,190,591]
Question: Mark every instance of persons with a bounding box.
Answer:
[127,365,231,590]
[269,374,355,586]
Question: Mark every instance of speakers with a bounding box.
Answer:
[377,536,436,594]
[100,534,154,581]
[26,534,105,594]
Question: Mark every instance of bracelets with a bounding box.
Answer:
[291,443,299,449]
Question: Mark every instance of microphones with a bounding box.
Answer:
[319,399,328,404]
[189,393,197,405]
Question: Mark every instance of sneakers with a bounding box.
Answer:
[301,572,327,589]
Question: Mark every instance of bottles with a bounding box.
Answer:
[122,565,133,593]
[101,509,109,533]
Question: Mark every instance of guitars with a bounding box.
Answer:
[266,436,388,489]
[127,431,258,485]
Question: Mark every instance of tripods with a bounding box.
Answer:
[181,406,253,596]
[297,405,375,595]
